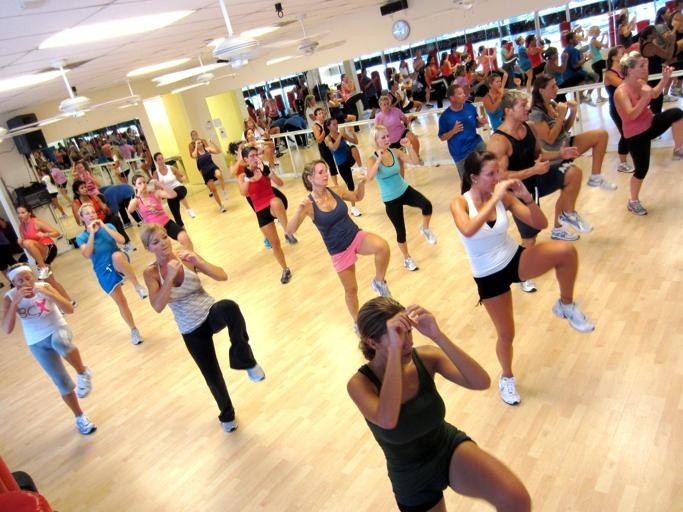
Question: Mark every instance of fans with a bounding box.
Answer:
[0,1,264,140]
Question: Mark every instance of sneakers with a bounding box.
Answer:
[220,420,237,431]
[518,211,593,294]
[580,94,608,104]
[420,226,436,245]
[221,190,227,199]
[617,161,634,174]
[285,234,297,243]
[627,198,646,214]
[264,240,271,247]
[75,416,97,435]
[425,103,433,107]
[248,365,265,380]
[372,279,391,297]
[358,168,366,175]
[220,205,226,212]
[131,328,142,345]
[77,368,91,398]
[350,207,361,217]
[188,209,195,217]
[136,286,147,298]
[209,191,213,196]
[498,375,520,405]
[586,174,616,191]
[281,268,290,283]
[672,149,681,160]
[122,243,136,253]
[403,258,418,271]
[553,300,595,332]
[662,85,682,101]
[37,267,51,280]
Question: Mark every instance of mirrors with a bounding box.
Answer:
[240,0,683,179]
[0,119,160,268]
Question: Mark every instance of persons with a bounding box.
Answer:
[603,1,683,216]
[189,129,230,213]
[0,263,97,437]
[140,222,266,434]
[33,128,195,346]
[283,160,391,341]
[230,0,617,292]
[345,296,530,512]
[0,201,79,314]
[448,150,595,407]
